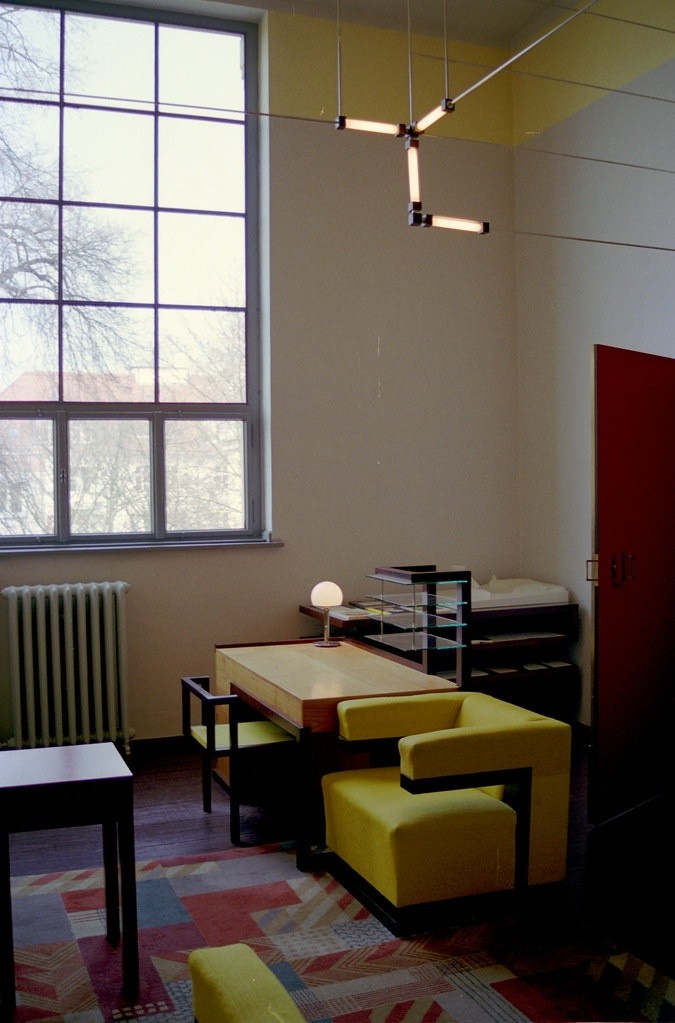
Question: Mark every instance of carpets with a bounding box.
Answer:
[0,802,675,1023]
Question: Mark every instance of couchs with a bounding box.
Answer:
[311,690,574,937]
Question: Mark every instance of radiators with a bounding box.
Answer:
[0,581,135,761]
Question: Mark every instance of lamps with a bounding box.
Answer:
[310,580,344,647]
[333,0,493,234]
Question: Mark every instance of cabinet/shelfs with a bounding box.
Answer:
[363,564,472,689]
[295,576,580,722]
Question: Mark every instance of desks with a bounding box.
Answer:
[0,742,139,1023]
[210,637,465,873]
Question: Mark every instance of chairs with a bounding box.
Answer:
[181,674,310,846]
